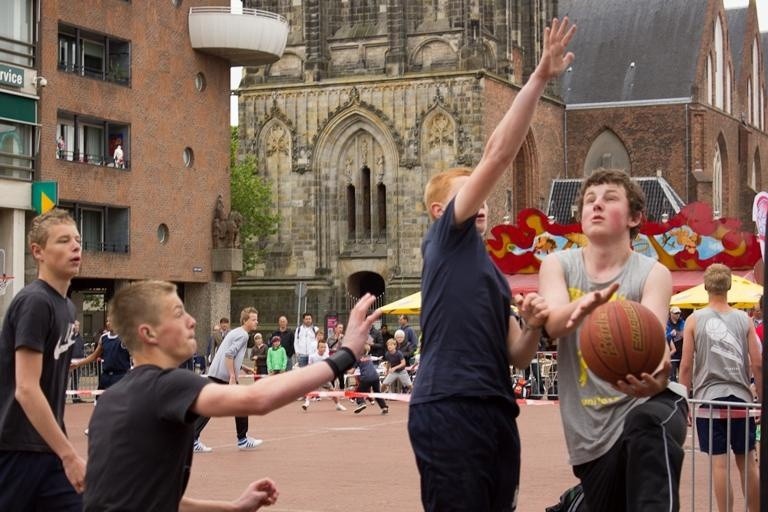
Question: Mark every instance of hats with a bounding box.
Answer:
[669,306,682,314]
[271,336,281,342]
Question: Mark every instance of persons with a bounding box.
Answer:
[266,312,417,413]
[0,206,91,510]
[514,310,558,400]
[251,333,268,375]
[70,316,130,436]
[754,296,764,345]
[535,167,692,509]
[666,308,685,380]
[193,306,262,453]
[406,13,584,510]
[81,280,384,510]
[678,262,764,510]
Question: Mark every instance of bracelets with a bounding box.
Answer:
[323,347,358,380]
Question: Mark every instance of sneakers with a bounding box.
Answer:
[336,405,346,411]
[192,436,213,453]
[353,403,367,413]
[237,438,262,450]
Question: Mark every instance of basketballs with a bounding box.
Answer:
[579,300,665,384]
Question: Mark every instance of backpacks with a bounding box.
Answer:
[512,377,532,398]
